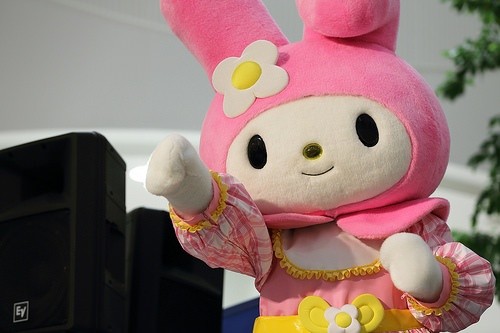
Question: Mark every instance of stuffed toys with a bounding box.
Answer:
[144,2,496,333]
